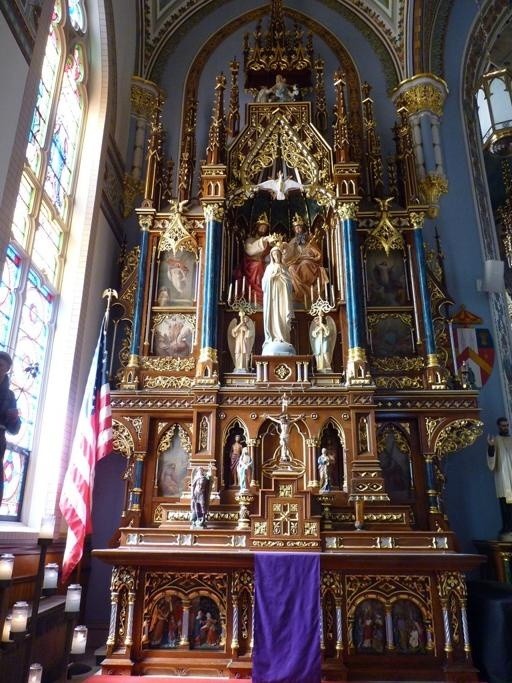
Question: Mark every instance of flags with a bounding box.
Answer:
[59,312,112,584]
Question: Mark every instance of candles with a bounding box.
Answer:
[0,515,87,682]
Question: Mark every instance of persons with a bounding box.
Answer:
[257,75,299,102]
[384,454,406,489]
[160,464,180,494]
[311,318,332,370]
[262,246,294,347]
[266,415,304,462]
[231,315,255,372]
[233,212,282,304]
[318,447,330,491]
[153,262,196,352]
[485,417,511,533]
[281,213,329,300]
[145,601,423,651]
[190,467,212,524]
[366,264,409,354]
[229,435,243,485]
[236,446,253,486]
[0,351,21,509]
